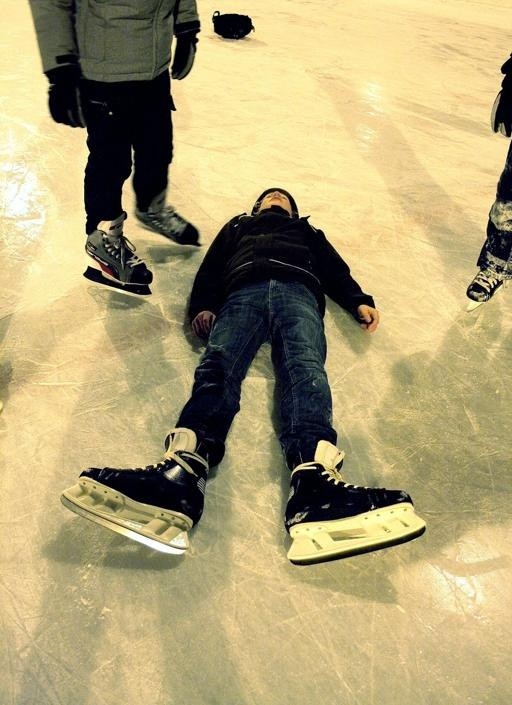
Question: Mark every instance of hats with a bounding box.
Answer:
[249,186,300,219]
[211,10,256,41]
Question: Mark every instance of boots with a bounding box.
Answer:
[84,211,153,286]
[76,426,209,530]
[283,439,415,537]
[134,191,199,246]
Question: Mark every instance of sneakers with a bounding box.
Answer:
[465,266,506,305]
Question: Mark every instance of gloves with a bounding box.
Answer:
[490,84,512,140]
[170,19,201,81]
[43,64,86,130]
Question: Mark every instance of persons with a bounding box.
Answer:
[466,46,512,302]
[30,0,199,287]
[81,188,413,535]
